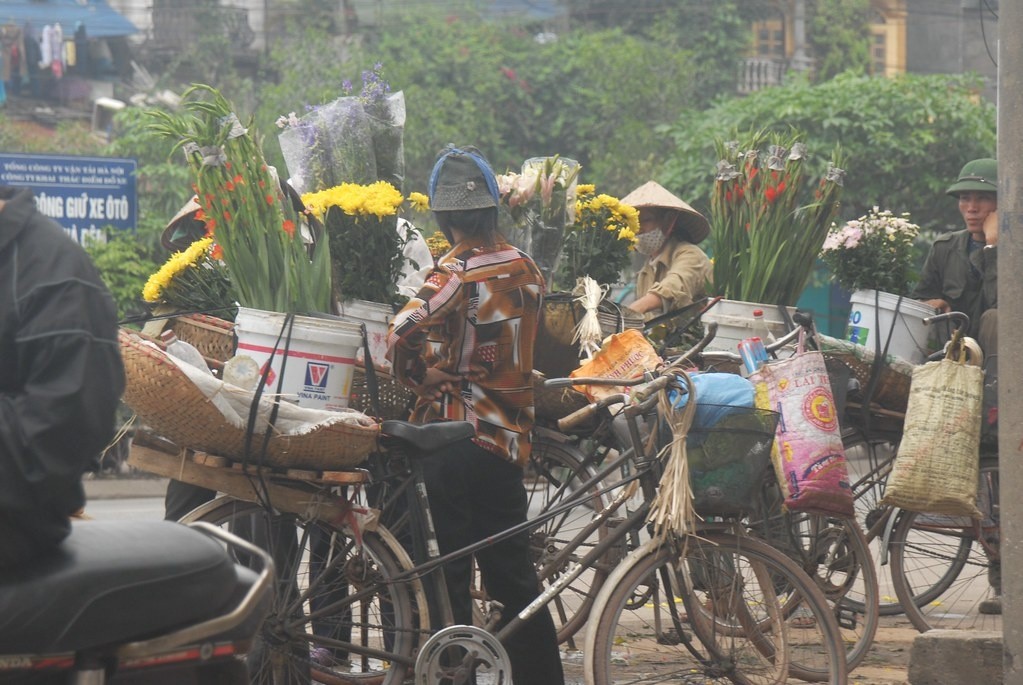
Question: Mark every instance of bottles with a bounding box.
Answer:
[223,353,260,391]
[160,328,213,385]
[752,308,774,348]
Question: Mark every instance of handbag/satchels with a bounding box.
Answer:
[882,332,984,516]
[746,324,856,519]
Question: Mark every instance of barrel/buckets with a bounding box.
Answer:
[544,290,580,346]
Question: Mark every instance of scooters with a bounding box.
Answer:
[0,514,280,685]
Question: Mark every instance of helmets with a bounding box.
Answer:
[945,158,997,199]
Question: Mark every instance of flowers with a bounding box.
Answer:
[823,206,918,296]
[561,183,638,294]
[277,67,407,198]
[142,84,331,317]
[497,151,581,290]
[142,235,238,323]
[708,125,847,306]
[302,180,428,302]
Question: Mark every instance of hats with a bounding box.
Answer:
[160,194,202,252]
[428,145,499,210]
[620,180,711,245]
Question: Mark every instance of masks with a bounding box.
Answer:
[631,221,671,256]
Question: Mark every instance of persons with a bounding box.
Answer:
[911,157,1003,613]
[301,211,447,685]
[613,181,818,628]
[388,145,566,685]
[0,187,126,578]
[159,172,353,664]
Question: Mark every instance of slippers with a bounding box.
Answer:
[309,648,352,670]
[790,608,817,628]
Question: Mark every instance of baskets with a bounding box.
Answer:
[650,402,781,516]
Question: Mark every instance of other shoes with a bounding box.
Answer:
[978,594,1003,616]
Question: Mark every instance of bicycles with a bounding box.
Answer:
[171,307,1002,685]
